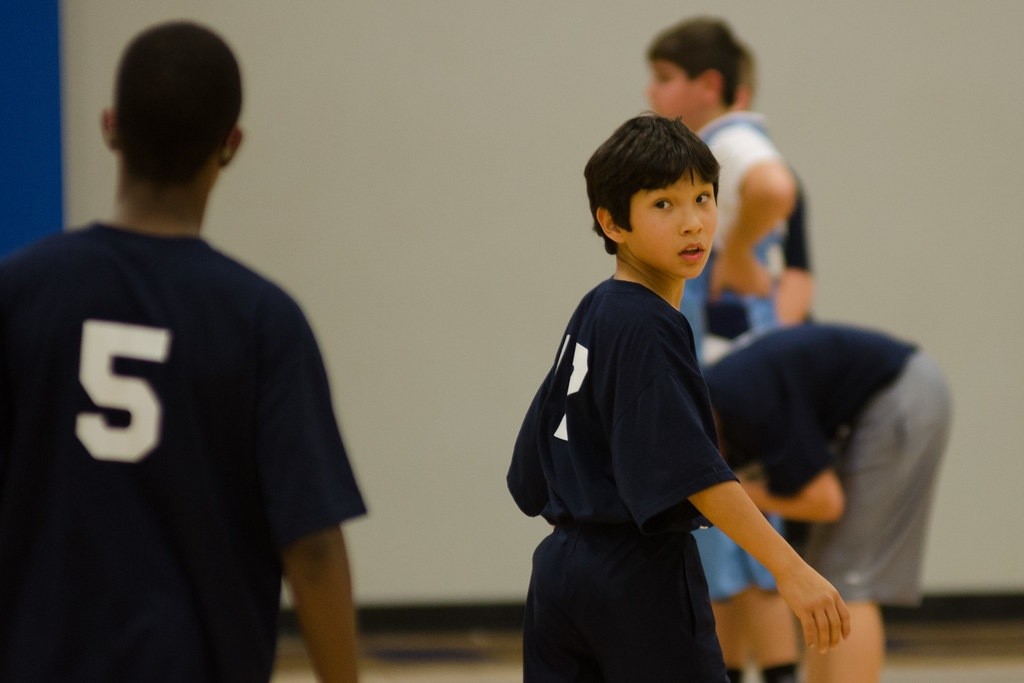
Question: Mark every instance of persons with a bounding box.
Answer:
[507,117,852,683]
[641,15,814,682]
[695,323,952,683]
[0,24,369,683]
